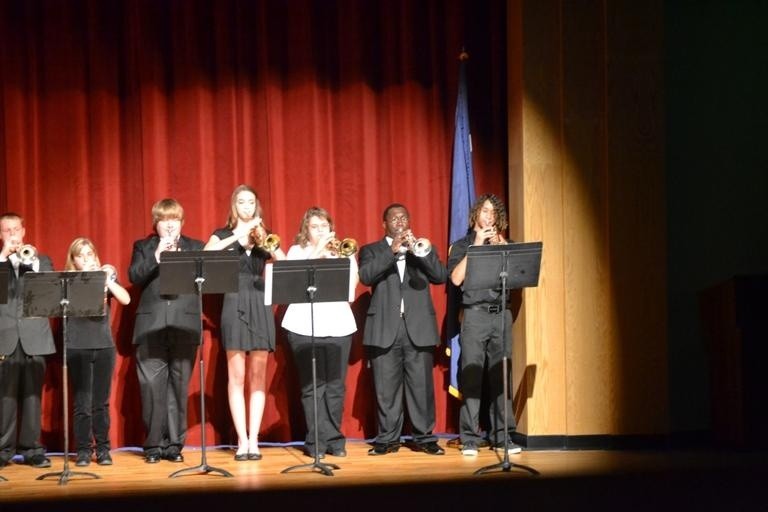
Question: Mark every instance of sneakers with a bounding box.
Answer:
[493,441,521,452]
[461,443,479,455]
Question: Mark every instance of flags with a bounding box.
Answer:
[448,53,476,402]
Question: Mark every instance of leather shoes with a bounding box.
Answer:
[75,449,91,467]
[165,447,183,463]
[248,450,264,460]
[30,452,52,468]
[234,453,246,461]
[415,440,444,456]
[146,451,161,464]
[306,448,325,459]
[367,442,401,454]
[97,449,112,465]
[327,445,349,458]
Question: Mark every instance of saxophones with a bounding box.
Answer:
[246,216,281,255]
[321,233,359,259]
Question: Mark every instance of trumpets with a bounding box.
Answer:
[10,238,39,266]
[89,263,118,289]
[395,227,433,259]
[483,221,503,246]
[163,231,180,253]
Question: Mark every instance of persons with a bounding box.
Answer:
[359,205,448,455]
[1,212,55,467]
[202,184,287,461]
[449,193,522,457]
[128,198,206,464]
[65,239,130,466]
[280,206,359,460]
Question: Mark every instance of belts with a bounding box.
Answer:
[463,304,512,313]
